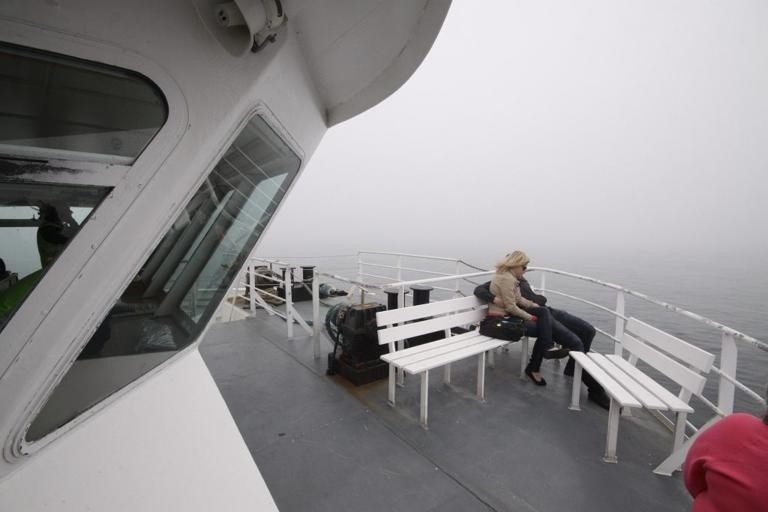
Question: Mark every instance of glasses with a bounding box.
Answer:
[523,265,526,271]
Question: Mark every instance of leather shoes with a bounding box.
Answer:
[526,368,546,385]
[543,348,571,359]
[588,391,621,414]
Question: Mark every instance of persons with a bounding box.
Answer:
[474,252,622,416]
[683,409,768,512]
[489,249,570,385]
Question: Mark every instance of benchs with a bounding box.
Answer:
[568,315,718,465]
[372,294,530,432]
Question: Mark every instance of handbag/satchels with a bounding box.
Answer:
[479,317,524,342]
